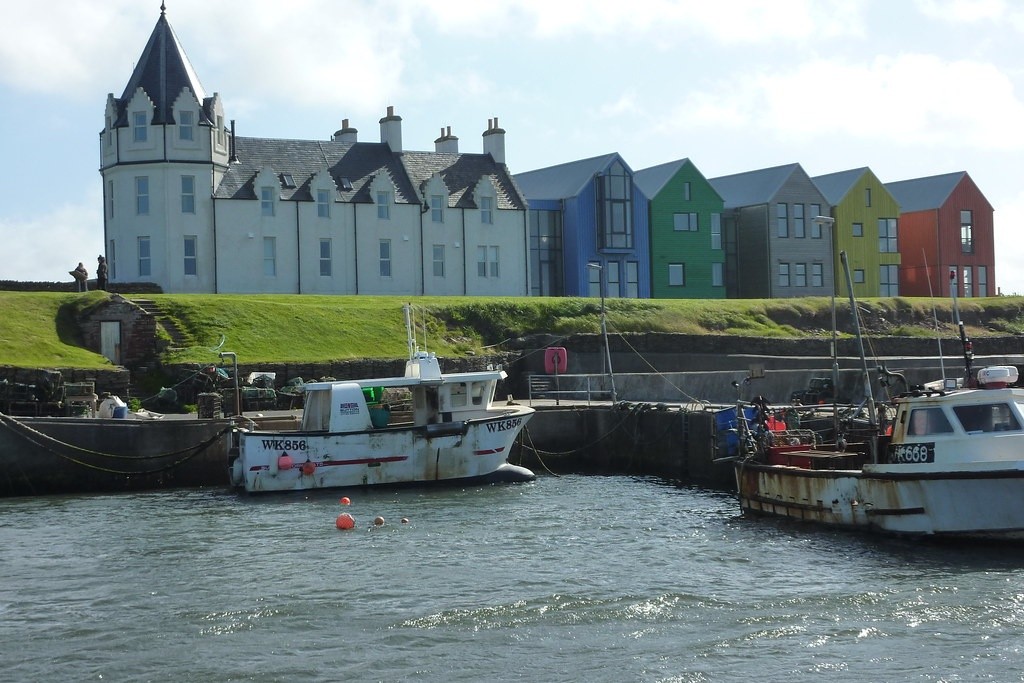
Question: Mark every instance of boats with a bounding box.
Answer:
[727,246,910,529]
[221,296,537,493]
[860,266,1024,548]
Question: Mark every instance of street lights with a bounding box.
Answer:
[814,216,840,446]
